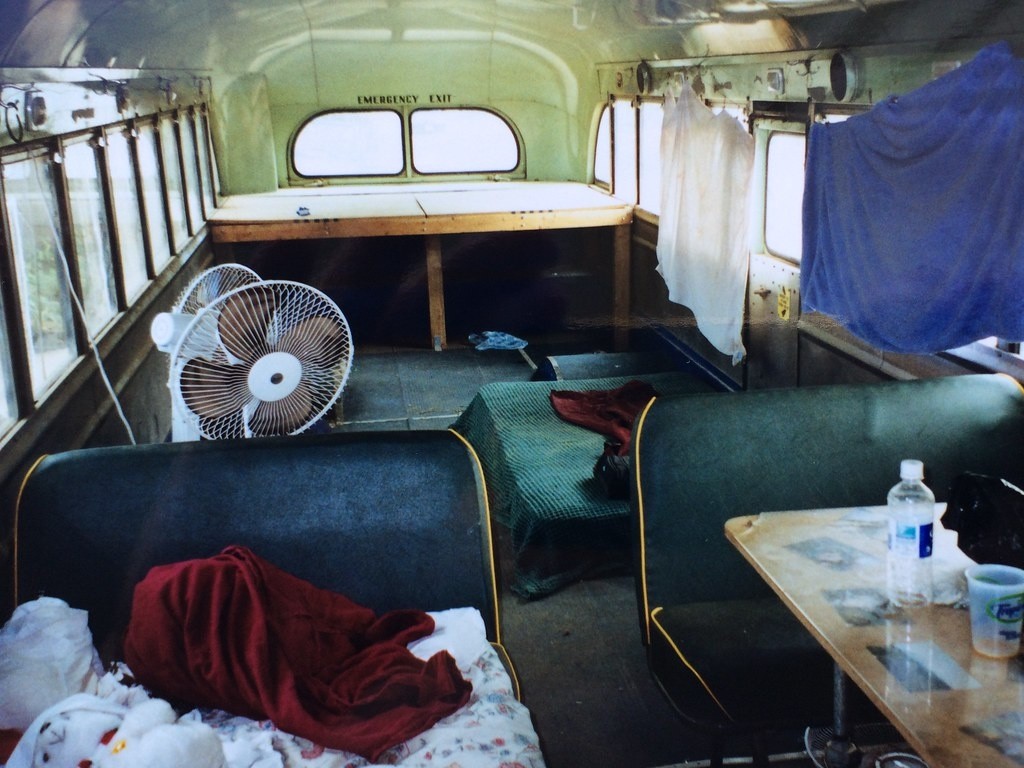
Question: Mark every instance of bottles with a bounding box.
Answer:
[887,460,936,610]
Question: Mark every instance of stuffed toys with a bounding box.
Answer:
[28,686,225,768]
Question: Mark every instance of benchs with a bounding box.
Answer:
[636,372,1024,729]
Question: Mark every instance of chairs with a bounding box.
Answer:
[16,428,518,699]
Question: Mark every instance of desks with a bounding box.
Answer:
[725,502,1024,768]
[207,182,632,352]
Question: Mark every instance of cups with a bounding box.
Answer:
[965,565,1024,657]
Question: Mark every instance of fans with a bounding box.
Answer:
[170,281,353,439]
[151,263,261,441]
